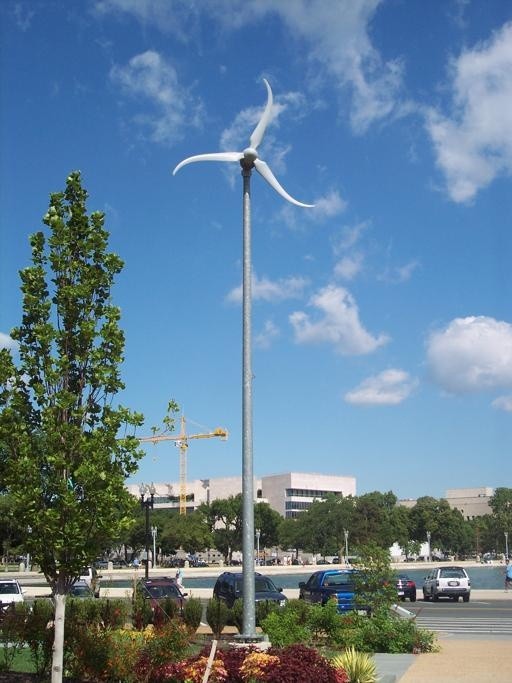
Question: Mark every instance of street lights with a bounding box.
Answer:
[344,527,350,564]
[151,525,160,569]
[138,479,155,579]
[504,532,510,561]
[255,528,261,564]
[425,530,433,563]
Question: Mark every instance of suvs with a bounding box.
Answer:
[420,566,472,603]
[212,569,293,628]
[132,576,189,624]
[299,565,380,618]
[1,577,26,611]
[75,564,103,599]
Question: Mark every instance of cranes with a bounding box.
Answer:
[121,415,232,519]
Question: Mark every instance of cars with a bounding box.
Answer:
[95,555,154,566]
[14,555,28,563]
[222,553,358,566]
[403,553,453,563]
[381,572,418,602]
[469,551,506,560]
[65,581,95,605]
[171,557,208,568]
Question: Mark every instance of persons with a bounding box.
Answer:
[173,564,185,590]
[475,551,506,564]
[502,558,512,593]
[134,557,139,571]
